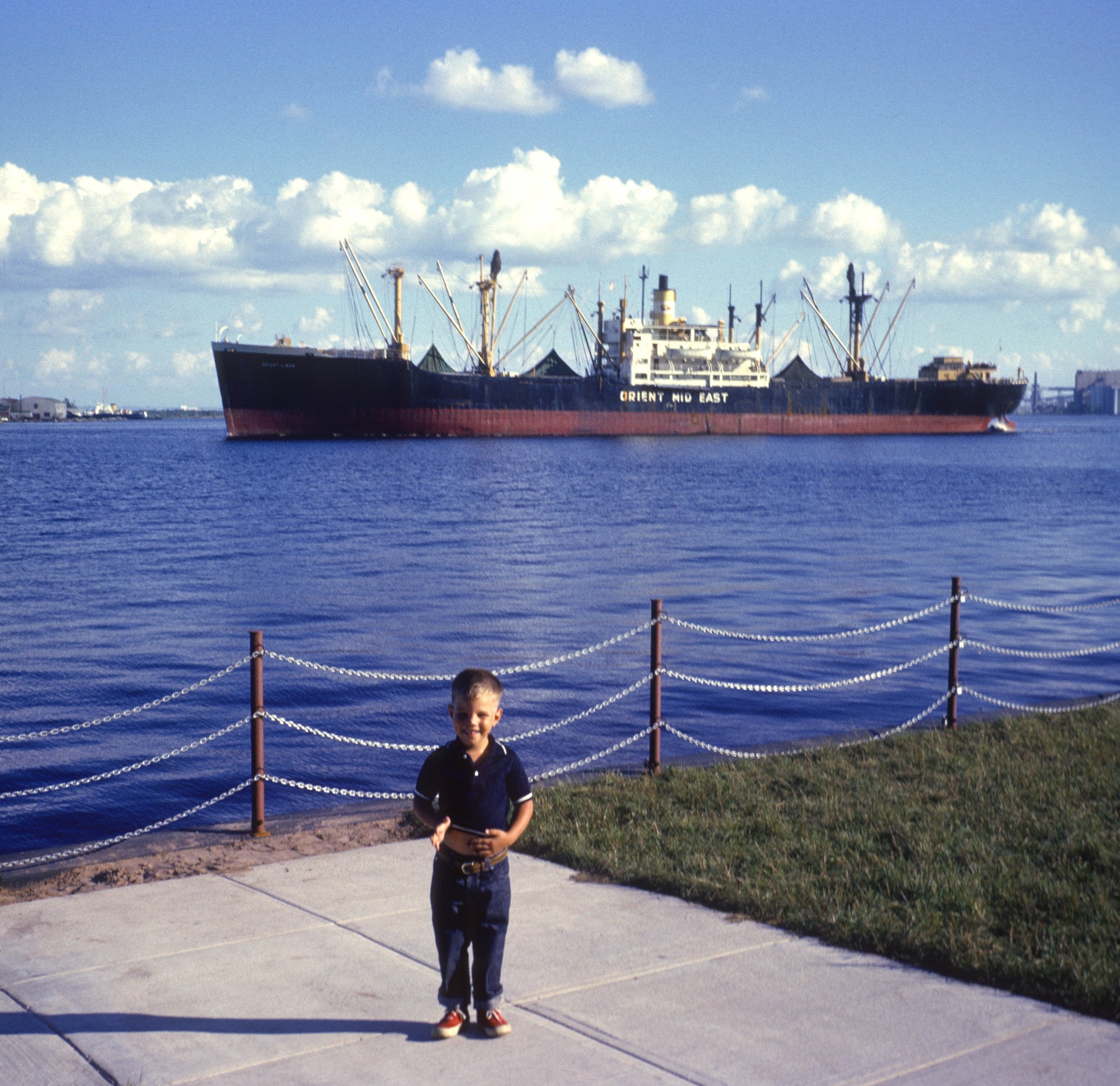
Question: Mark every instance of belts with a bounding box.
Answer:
[436,846,507,875]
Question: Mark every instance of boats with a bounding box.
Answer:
[212,237,1029,443]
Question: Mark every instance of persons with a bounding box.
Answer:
[413,669,533,1038]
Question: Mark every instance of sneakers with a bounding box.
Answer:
[476,1008,511,1037]
[432,1008,470,1038]
